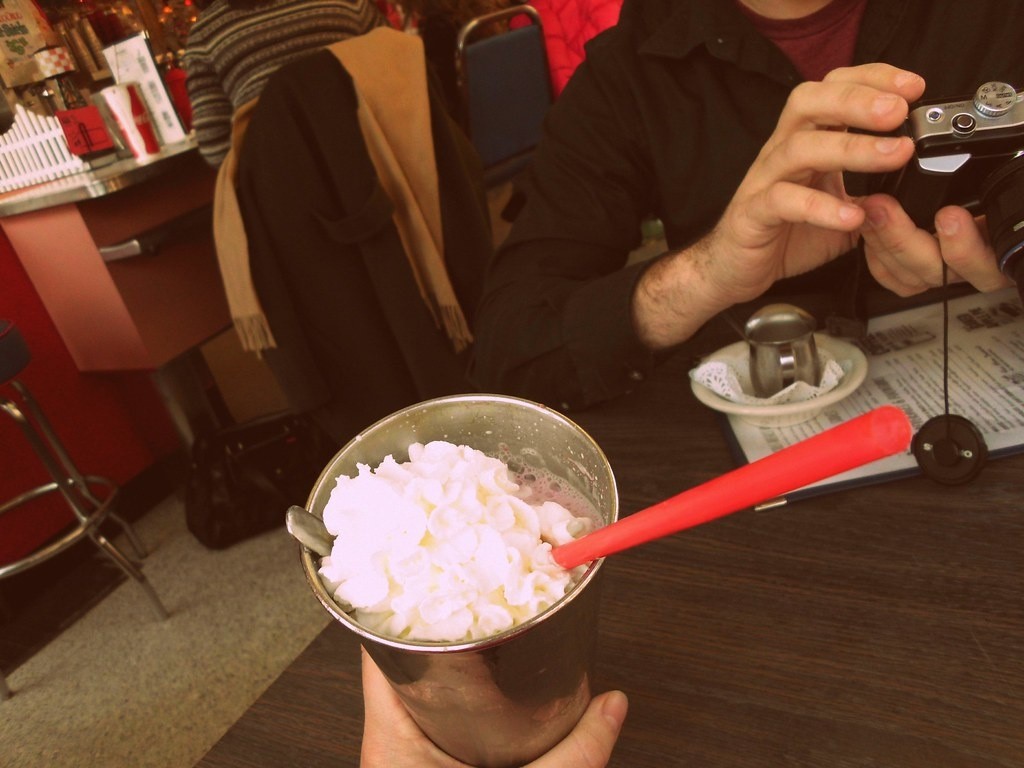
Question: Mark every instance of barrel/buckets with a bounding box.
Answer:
[88,79,163,163]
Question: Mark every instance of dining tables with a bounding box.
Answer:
[191,306,1023,767]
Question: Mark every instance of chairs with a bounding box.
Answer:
[438,6,557,251]
[0,318,171,702]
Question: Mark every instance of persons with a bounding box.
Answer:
[471,3,1023,417]
[356,644,629,766]
[182,1,398,176]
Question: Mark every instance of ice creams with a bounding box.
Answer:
[303,441,600,652]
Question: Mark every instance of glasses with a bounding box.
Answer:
[827,309,870,369]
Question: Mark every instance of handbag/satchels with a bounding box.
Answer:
[184,406,320,549]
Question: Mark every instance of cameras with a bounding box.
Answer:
[862,82,1024,300]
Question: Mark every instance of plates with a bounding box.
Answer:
[692,334,868,428]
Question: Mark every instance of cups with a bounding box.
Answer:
[299,394,620,768]
[743,301,822,403]
